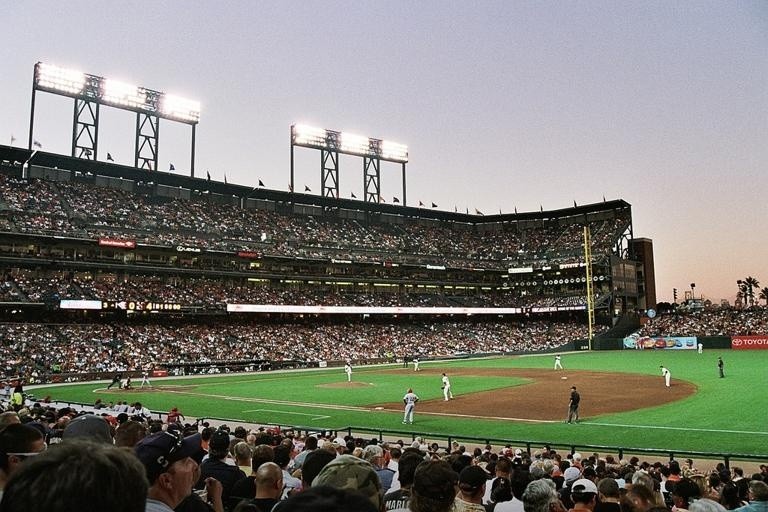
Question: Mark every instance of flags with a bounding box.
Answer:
[107,153,114,161]
[34,139,41,148]
[169,163,177,170]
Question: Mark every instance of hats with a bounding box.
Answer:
[134,431,202,485]
[460,465,492,492]
[209,431,230,459]
[563,467,598,495]
[63,414,112,445]
[665,478,700,498]
[311,454,384,510]
[413,459,456,502]
[332,437,349,452]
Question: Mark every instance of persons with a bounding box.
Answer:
[440,372,454,402]
[1,171,630,389]
[401,388,419,425]
[697,342,703,354]
[660,366,671,388]
[1,390,183,511]
[184,418,767,512]
[564,386,580,424]
[717,357,725,378]
[633,304,768,350]
